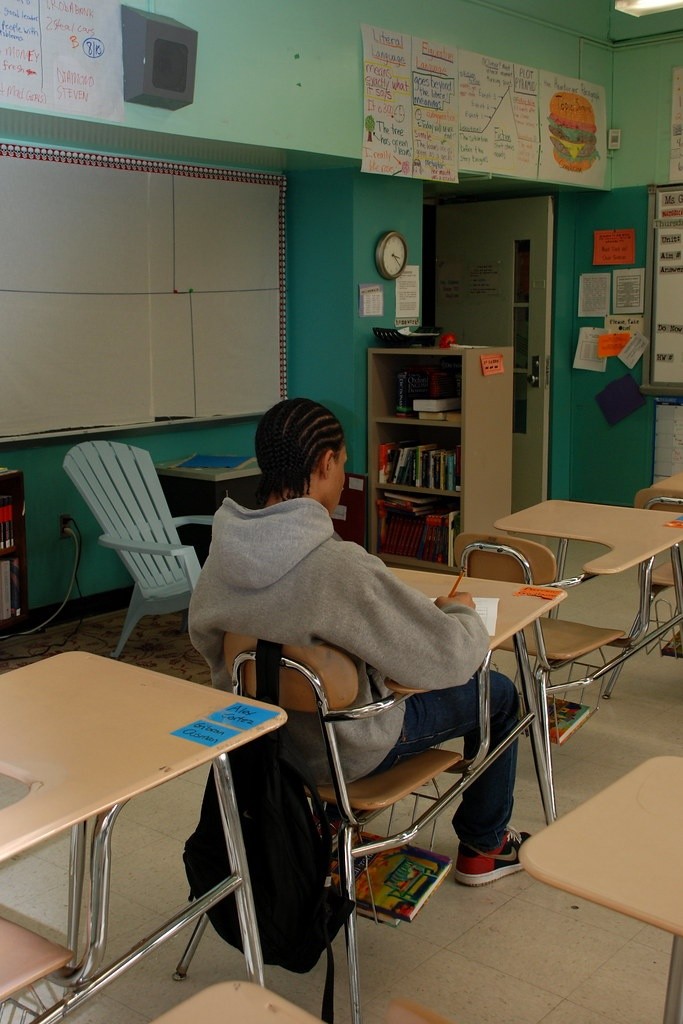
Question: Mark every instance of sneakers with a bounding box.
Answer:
[455,824,533,886]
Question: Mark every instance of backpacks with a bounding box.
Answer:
[182,639,355,974]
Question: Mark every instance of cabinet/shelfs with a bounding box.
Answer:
[368,347,513,573]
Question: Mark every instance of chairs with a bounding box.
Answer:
[63,442,221,659]
[602,489,682,700]
[431,534,623,825]
[146,623,464,1023]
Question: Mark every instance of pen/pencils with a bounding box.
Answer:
[447,566,468,599]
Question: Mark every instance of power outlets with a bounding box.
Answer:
[59,512,75,541]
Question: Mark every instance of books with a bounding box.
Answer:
[327,831,453,928]
[0,495,21,620]
[534,698,594,744]
[377,365,461,567]
[663,630,683,659]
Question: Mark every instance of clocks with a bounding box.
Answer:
[375,232,408,281]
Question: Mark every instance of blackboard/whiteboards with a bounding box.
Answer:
[639,184,683,397]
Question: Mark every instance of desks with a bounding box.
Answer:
[518,755,682,1024]
[492,498,683,698]
[361,567,568,830]
[0,650,287,986]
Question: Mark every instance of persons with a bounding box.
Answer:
[187,398,533,887]
